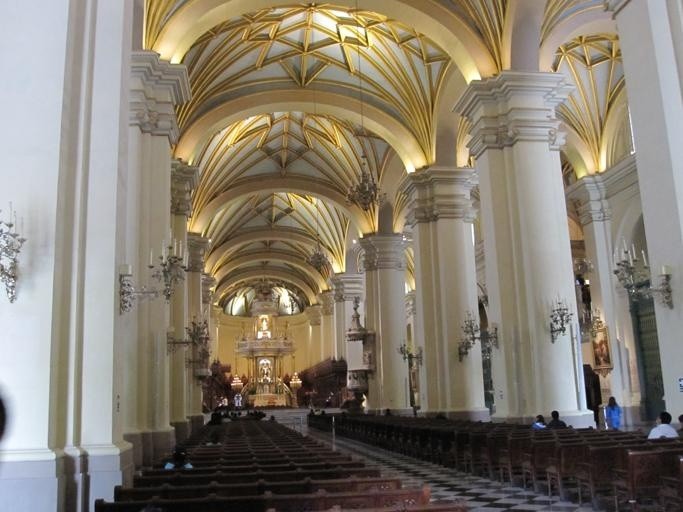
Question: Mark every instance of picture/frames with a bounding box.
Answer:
[587,324,613,378]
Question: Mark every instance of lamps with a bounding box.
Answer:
[577,307,603,338]
[0,200,28,304]
[546,294,572,340]
[116,240,188,316]
[165,316,213,369]
[395,341,427,365]
[613,236,675,308]
[457,310,502,362]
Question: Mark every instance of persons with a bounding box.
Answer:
[675,414,683,438]
[545,410,567,428]
[204,434,222,446]
[583,363,602,427]
[646,411,678,438]
[319,410,326,414]
[384,407,393,415]
[165,448,193,470]
[209,406,275,423]
[308,408,314,415]
[530,414,547,430]
[605,395,623,432]
